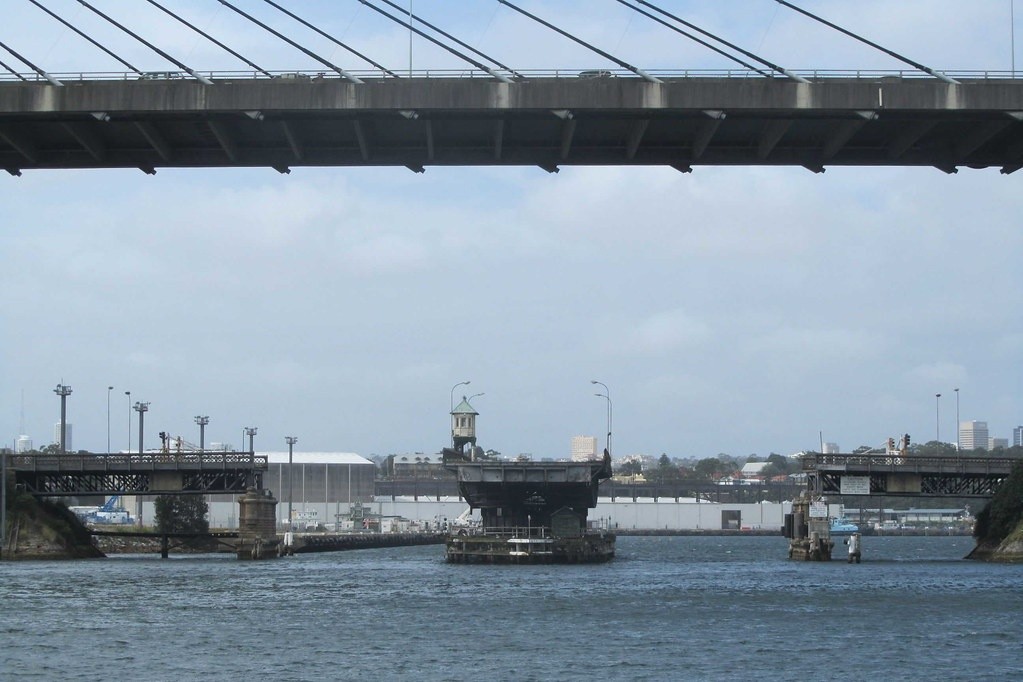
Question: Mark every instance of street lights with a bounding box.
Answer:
[591,381,609,452]
[467,393,485,403]
[450,382,470,448]
[936,394,941,456]
[954,389,959,450]
[108,387,113,453]
[595,394,612,456]
[126,392,130,453]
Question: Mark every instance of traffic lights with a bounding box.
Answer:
[906,434,910,445]
[892,442,895,447]
[159,432,165,443]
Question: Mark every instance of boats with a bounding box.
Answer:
[445,448,616,565]
